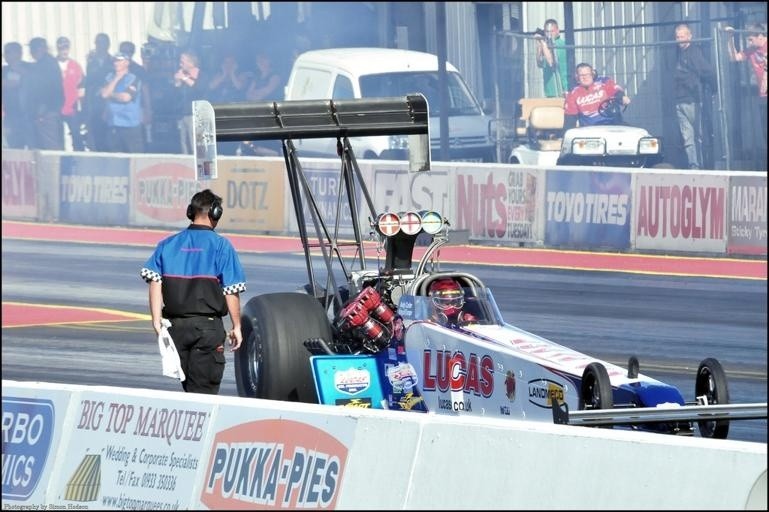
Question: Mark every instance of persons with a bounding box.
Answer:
[564,63,631,128]
[2,33,282,155]
[674,24,715,169]
[535,19,568,97]
[140,189,247,394]
[725,22,767,140]
[428,278,475,322]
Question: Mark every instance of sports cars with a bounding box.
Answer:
[190,92,730,441]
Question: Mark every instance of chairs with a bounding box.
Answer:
[514,97,566,152]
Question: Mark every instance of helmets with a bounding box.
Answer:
[428,278,465,315]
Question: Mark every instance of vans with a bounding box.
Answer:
[283,46,497,163]
